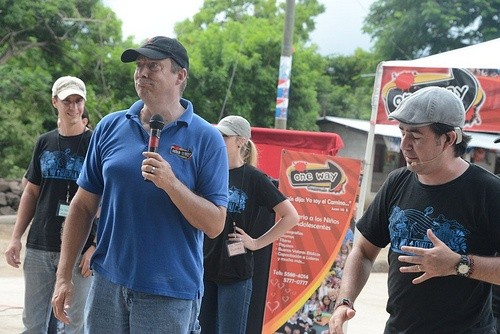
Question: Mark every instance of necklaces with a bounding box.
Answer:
[57,125,86,190]
[227,163,245,227]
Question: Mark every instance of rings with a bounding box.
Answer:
[151,167,156,173]
[418,265,422,272]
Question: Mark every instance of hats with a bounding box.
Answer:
[215,115,250,138]
[388,86,465,129]
[52,76,86,101]
[121,36,188,71]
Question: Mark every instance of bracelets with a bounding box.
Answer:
[468,255,474,278]
[91,242,96,248]
[333,298,353,311]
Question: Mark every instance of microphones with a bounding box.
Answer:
[143,113,165,182]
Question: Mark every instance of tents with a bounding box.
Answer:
[355,35,500,225]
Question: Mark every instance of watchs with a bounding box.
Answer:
[455,255,472,278]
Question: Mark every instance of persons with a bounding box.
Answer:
[198,115,300,334]
[5,75,104,334]
[285,202,358,334]
[328,85,500,334]
[51,36,230,334]
